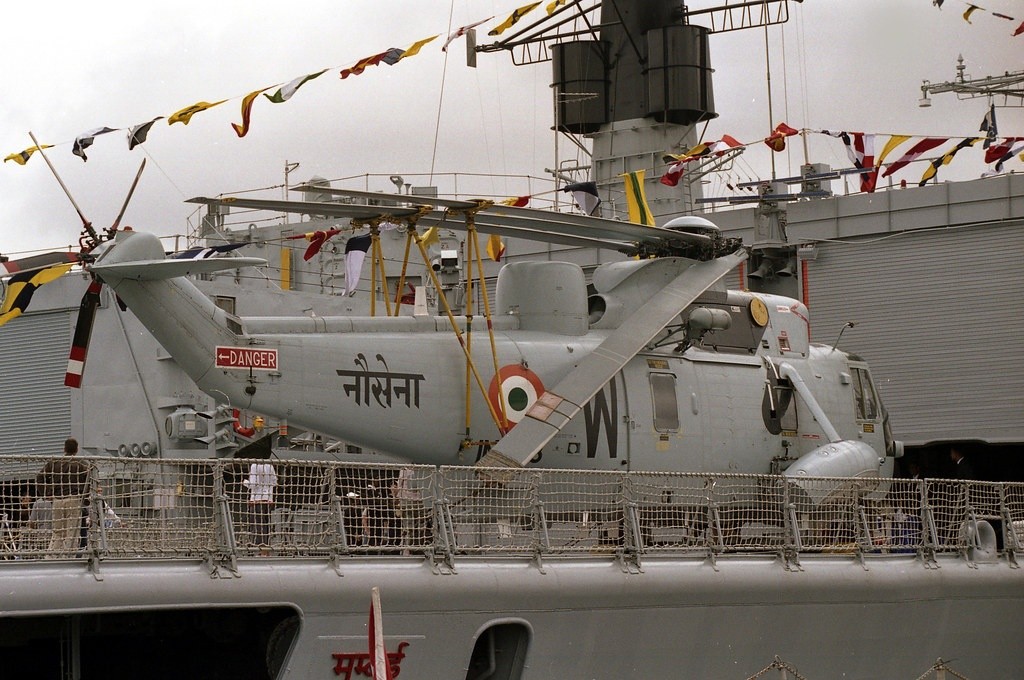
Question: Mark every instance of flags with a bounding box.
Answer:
[0,0,1024,328]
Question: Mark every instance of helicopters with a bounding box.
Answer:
[85,185,910,551]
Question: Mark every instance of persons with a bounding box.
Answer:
[895,449,982,546]
[11,440,437,562]
